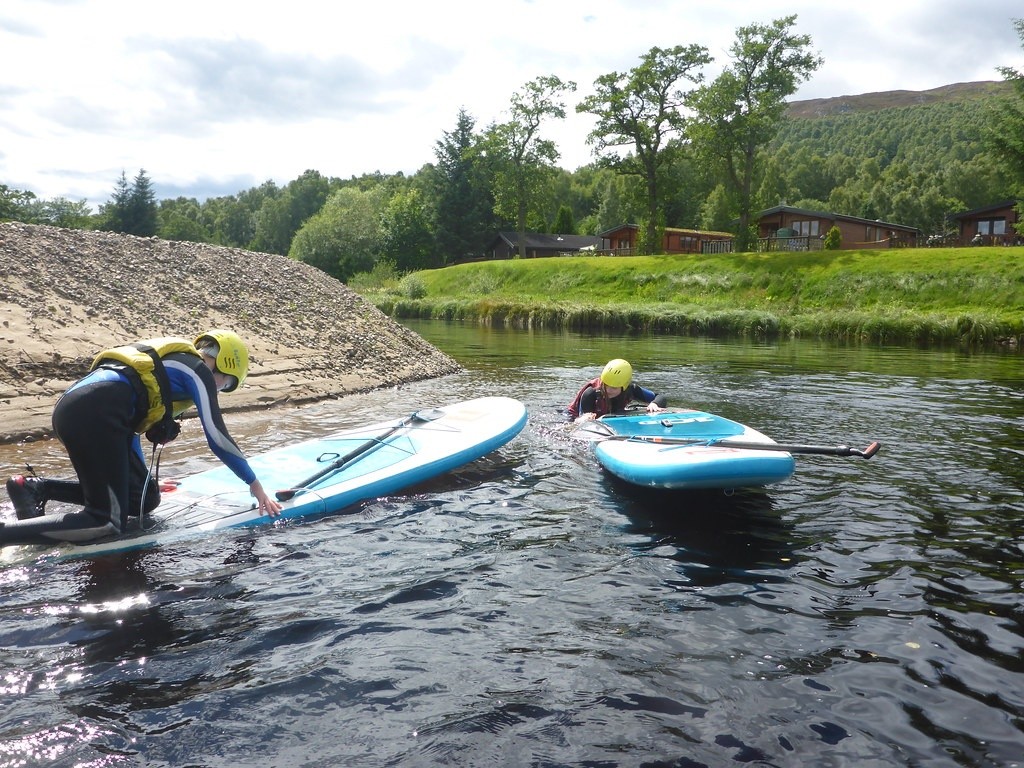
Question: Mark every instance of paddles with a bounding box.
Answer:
[569,415,880,461]
[276,407,446,500]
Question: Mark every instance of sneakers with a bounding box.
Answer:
[6,475,47,520]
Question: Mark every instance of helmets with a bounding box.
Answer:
[193,329,248,392]
[601,358,632,391]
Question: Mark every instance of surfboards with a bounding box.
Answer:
[579,408,795,490]
[0,391,528,562]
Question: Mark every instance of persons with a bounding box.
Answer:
[0,330,283,548]
[558,358,667,423]
[1013,232,1019,246]
